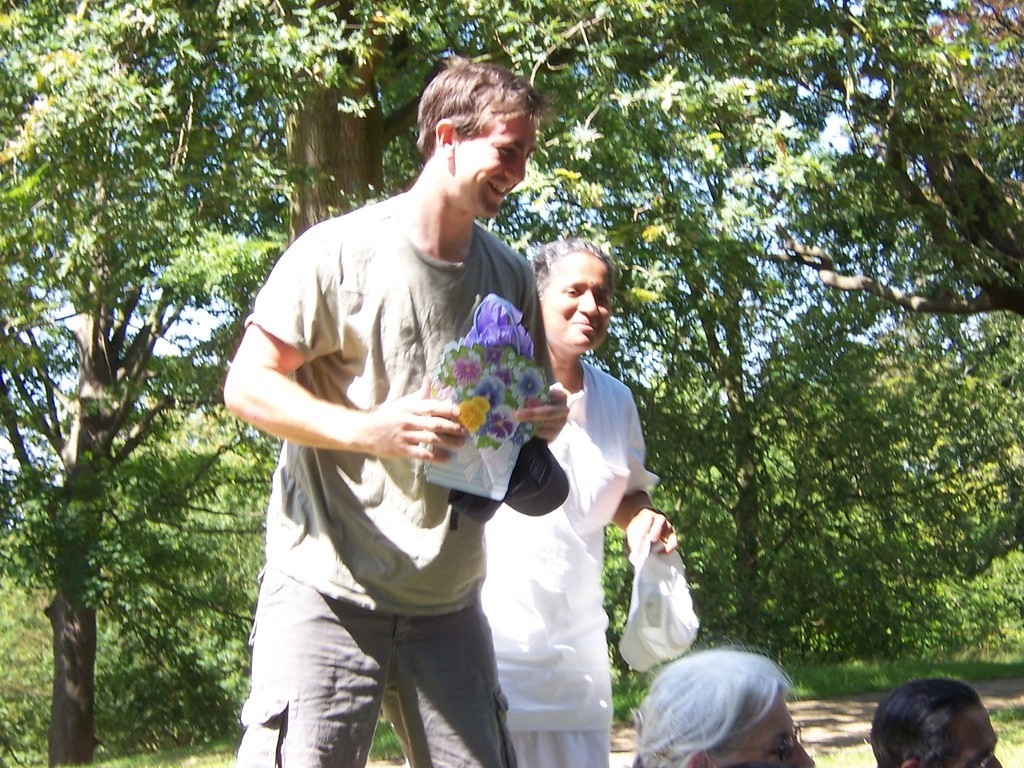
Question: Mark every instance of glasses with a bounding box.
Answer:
[979,741,999,768]
[729,724,803,762]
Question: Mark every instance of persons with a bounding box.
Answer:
[223,61,569,768]
[869,677,1002,768]
[632,648,817,767]
[480,239,677,767]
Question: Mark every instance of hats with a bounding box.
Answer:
[619,536,699,672]
